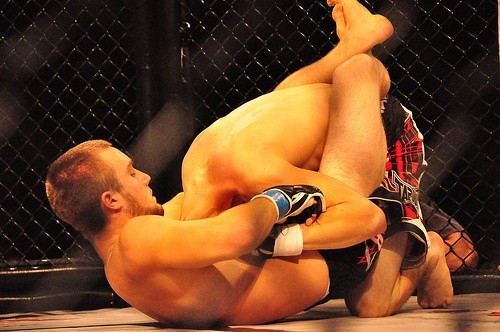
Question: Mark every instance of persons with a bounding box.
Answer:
[178,82,456,318]
[44,0,396,329]
[418,189,479,296]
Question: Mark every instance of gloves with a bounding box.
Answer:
[250,185,326,226]
[251,224,303,257]
